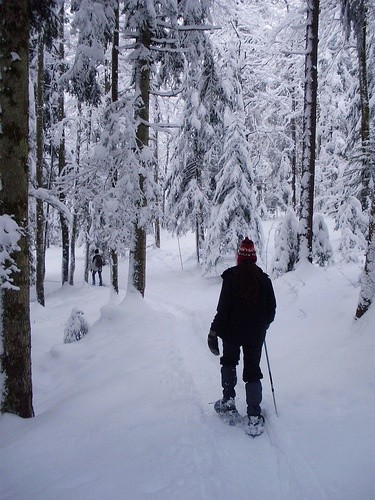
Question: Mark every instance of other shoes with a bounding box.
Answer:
[220,404,237,416]
[248,415,259,427]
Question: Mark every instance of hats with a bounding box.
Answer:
[237,237,257,264]
[95,249,99,253]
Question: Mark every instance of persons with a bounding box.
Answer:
[207,237,277,436]
[90,249,104,286]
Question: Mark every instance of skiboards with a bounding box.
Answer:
[208,399,265,439]
